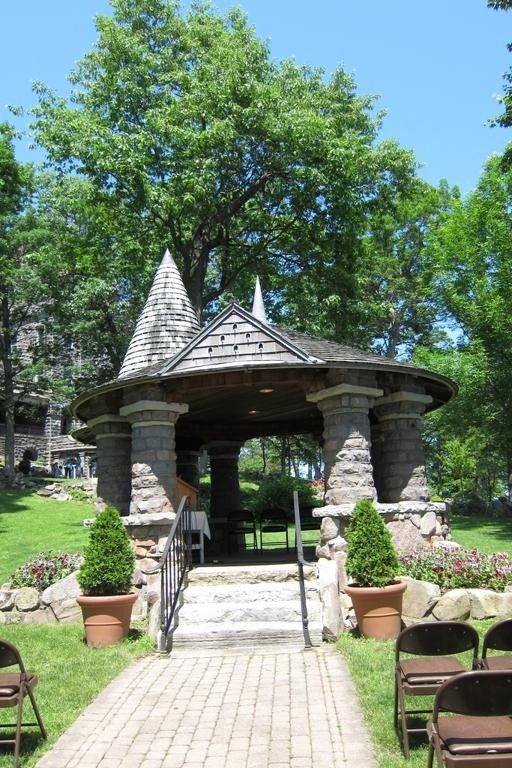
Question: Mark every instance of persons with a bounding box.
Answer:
[50,455,97,479]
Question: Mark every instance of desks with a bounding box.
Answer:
[180,511,212,564]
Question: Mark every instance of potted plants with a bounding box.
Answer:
[77,503,138,647]
[342,496,406,641]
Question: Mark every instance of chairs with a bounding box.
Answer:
[260,508,290,553]
[424,668,511,768]
[293,505,322,551]
[391,621,479,760]
[0,636,47,767]
[227,509,257,554]
